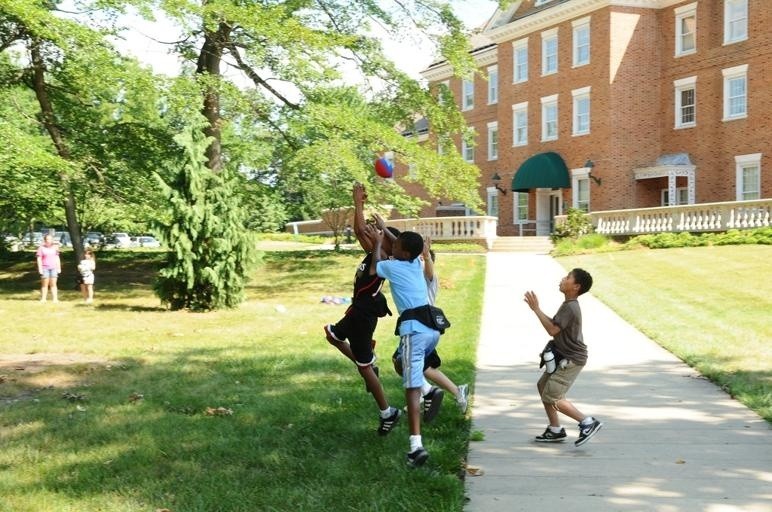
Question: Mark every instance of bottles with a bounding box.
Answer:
[274,303,286,314]
[543,346,557,374]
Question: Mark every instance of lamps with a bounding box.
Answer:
[492,170,507,196]
[584,159,603,187]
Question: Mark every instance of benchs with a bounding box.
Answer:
[518,219,554,236]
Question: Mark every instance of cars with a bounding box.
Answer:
[0,228,160,254]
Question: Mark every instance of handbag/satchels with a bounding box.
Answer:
[539,341,566,371]
[398,305,451,334]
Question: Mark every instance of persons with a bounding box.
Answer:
[525,268,603,446]
[37,234,62,304]
[391,237,468,414]
[326,182,403,437]
[365,213,444,467]
[78,251,96,302]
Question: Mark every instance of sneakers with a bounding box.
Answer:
[379,406,402,436]
[423,385,443,423]
[459,385,469,412]
[574,418,602,447]
[535,427,567,442]
[406,448,429,470]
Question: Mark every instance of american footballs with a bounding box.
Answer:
[372,159,392,178]
